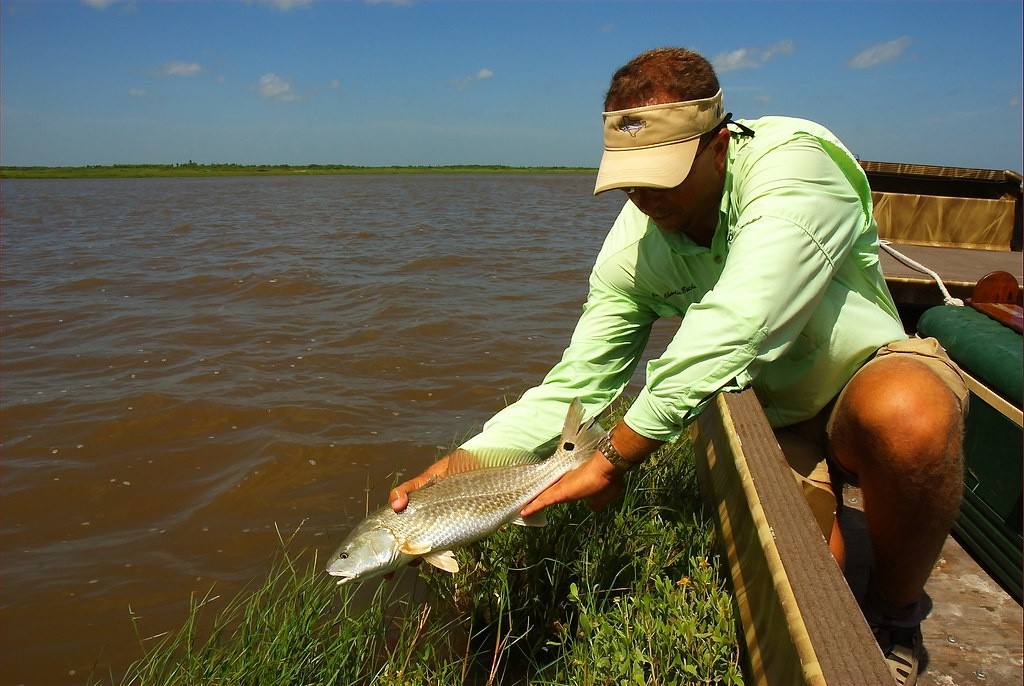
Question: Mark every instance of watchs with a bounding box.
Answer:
[596,425,643,471]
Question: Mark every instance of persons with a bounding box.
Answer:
[389,47,969,686]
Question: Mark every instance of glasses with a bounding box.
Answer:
[620,113,732,194]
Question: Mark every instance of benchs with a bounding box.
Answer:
[870,191,1024,303]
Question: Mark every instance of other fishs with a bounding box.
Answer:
[323,397,610,589]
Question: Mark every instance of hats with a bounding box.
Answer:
[593,88,727,196]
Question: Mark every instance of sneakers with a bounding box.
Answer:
[867,589,934,686]
